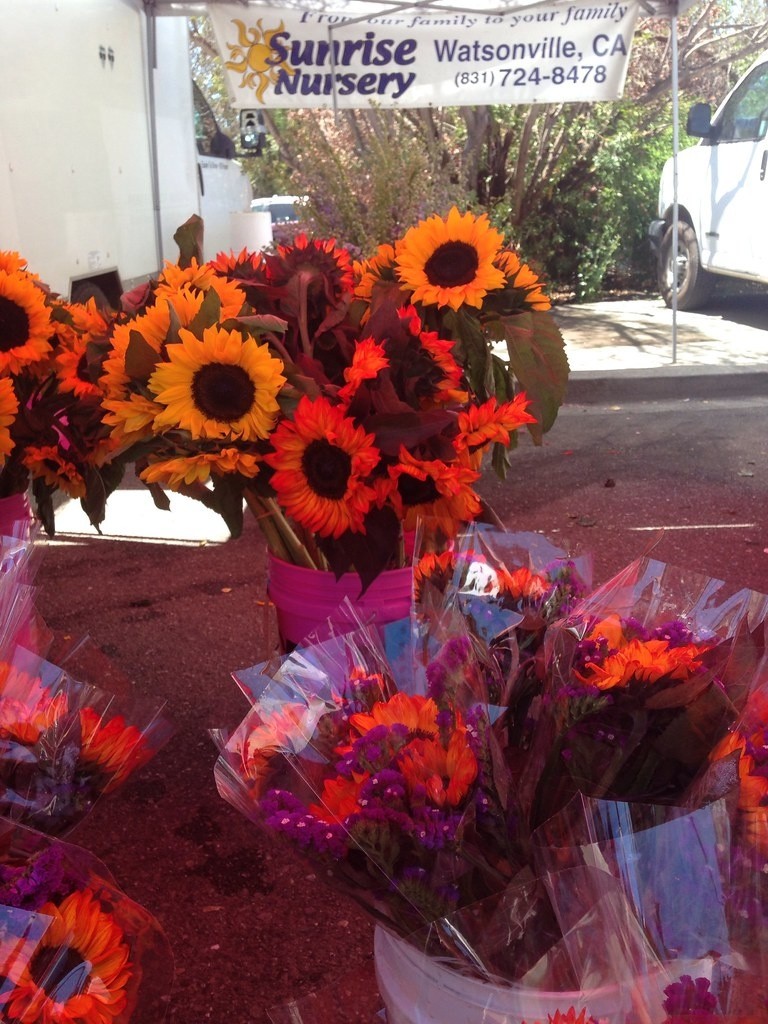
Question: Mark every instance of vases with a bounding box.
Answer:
[264,551,417,651]
[370,925,714,1023]
[0,491,40,653]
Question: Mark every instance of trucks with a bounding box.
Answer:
[1,1,275,312]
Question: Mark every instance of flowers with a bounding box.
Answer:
[89,202,577,567]
[1,249,768,1024]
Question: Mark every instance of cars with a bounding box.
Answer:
[249,197,313,242]
[650,46,767,308]
